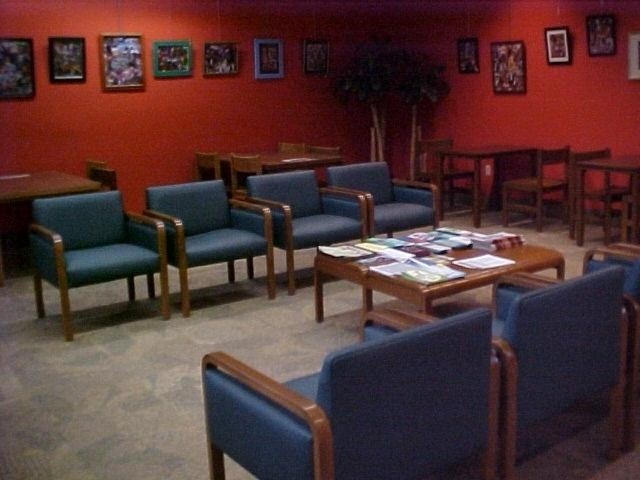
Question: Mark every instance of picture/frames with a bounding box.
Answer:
[303,39,330,75]
[48,37,85,82]
[152,39,193,78]
[254,39,285,79]
[0,38,35,98]
[202,40,240,75]
[457,13,640,95]
[99,32,146,91]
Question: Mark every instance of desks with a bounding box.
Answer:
[0,169,102,264]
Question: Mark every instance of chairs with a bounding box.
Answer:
[388,266,628,480]
[413,139,640,246]
[511,247,640,452]
[608,241,640,254]
[200,309,500,480]
[87,141,342,193]
[141,181,276,317]
[324,161,440,238]
[243,172,367,295]
[29,191,172,342]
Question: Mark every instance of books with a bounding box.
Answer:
[318,228,527,285]
